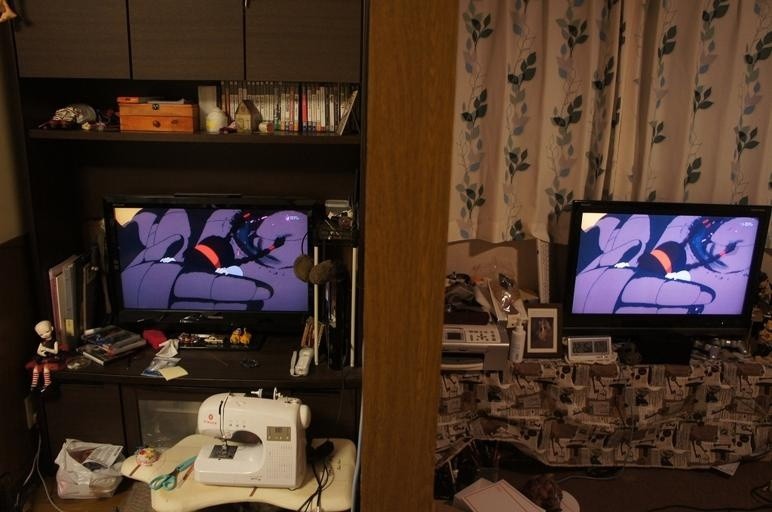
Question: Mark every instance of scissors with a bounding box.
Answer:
[149,456,197,491]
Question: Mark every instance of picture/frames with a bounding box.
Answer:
[524,302,563,358]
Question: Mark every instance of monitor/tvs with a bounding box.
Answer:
[557,198,772,365]
[100,192,324,352]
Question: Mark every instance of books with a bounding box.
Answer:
[48,255,139,368]
[198,81,353,133]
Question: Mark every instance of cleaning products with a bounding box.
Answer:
[510,318,528,363]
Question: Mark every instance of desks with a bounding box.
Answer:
[438,357,772,471]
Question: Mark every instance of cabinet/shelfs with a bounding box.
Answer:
[1,0,367,463]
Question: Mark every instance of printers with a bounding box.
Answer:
[439,308,510,373]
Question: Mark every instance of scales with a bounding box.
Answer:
[442,321,510,354]
